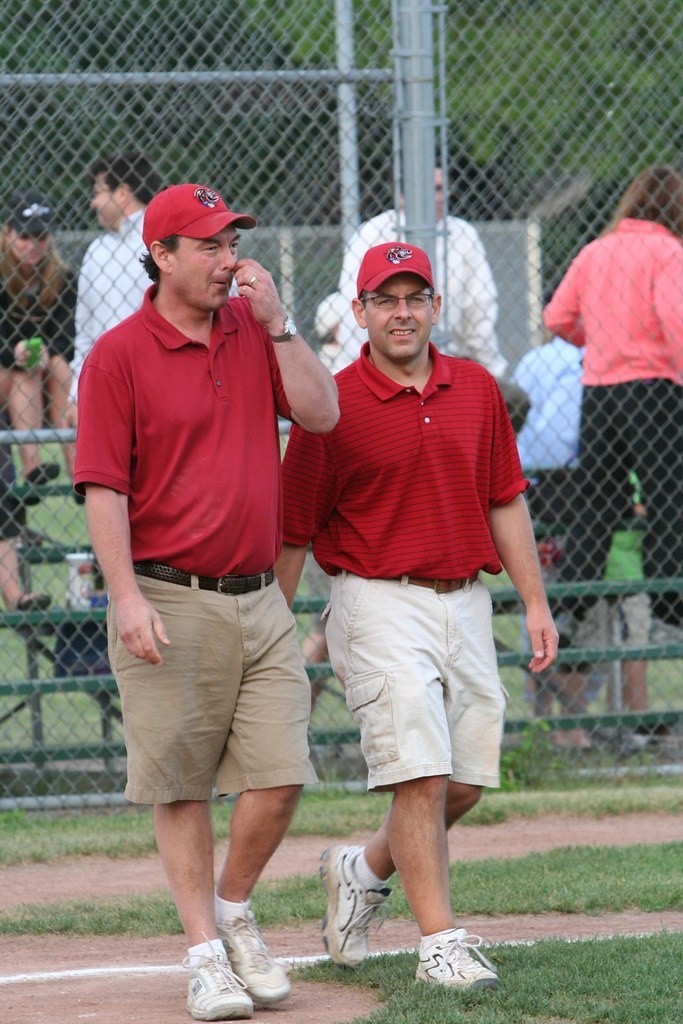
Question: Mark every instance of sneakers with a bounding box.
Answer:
[415,927,501,990]
[217,899,289,1003]
[181,930,253,1020]
[318,844,391,968]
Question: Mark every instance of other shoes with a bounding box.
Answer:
[16,593,50,611]
[21,461,61,507]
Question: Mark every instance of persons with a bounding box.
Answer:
[73,184,342,1018]
[514,165,683,747]
[275,242,558,990]
[0,154,241,612]
[303,164,507,708]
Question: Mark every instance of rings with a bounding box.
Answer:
[249,276,255,286]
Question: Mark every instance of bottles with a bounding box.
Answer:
[26,337,42,368]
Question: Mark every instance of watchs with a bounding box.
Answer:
[264,313,296,343]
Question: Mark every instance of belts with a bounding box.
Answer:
[395,575,476,595]
[133,563,276,596]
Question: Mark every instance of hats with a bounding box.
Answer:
[0,193,53,233]
[141,183,256,250]
[356,243,434,298]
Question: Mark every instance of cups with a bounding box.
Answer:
[65,553,95,607]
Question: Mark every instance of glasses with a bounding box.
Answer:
[362,294,433,309]
